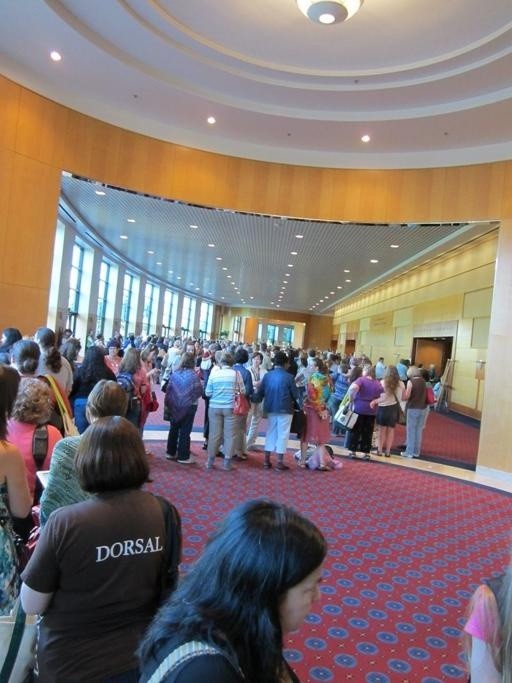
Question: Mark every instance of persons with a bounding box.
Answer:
[3,378,65,500]
[428,363,436,379]
[376,357,386,377]
[305,347,375,365]
[73,347,117,435]
[329,367,349,438]
[9,340,73,438]
[297,362,335,474]
[463,561,512,683]
[104,342,123,378]
[140,345,161,387]
[40,379,127,534]
[230,349,253,459]
[256,352,304,471]
[376,366,405,458]
[398,359,408,379]
[133,496,327,683]
[156,334,230,353]
[20,416,183,683]
[0,328,22,367]
[418,363,425,377]
[86,330,94,347]
[95,334,104,347]
[205,353,246,471]
[245,352,268,451]
[163,353,203,465]
[0,367,32,616]
[58,338,81,372]
[344,365,387,460]
[231,342,302,352]
[107,330,155,348]
[116,348,151,455]
[400,366,426,459]
[32,328,73,400]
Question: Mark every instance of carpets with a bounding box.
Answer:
[143,383,481,471]
[142,439,512,683]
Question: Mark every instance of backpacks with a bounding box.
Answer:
[117,372,138,412]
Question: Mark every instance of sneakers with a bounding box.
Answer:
[238,453,248,460]
[413,453,419,458]
[222,458,232,471]
[377,450,382,456]
[205,456,215,469]
[349,452,356,458]
[275,462,289,471]
[166,453,177,459]
[385,451,390,457]
[177,455,194,464]
[362,455,370,460]
[298,462,306,468]
[263,462,272,469]
[400,451,413,458]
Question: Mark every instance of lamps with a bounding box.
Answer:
[297,0,364,26]
[475,360,485,414]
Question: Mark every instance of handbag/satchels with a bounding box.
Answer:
[393,390,406,425]
[232,387,249,416]
[333,400,359,430]
[160,363,172,392]
[44,373,80,437]
[426,382,434,404]
[289,409,306,434]
